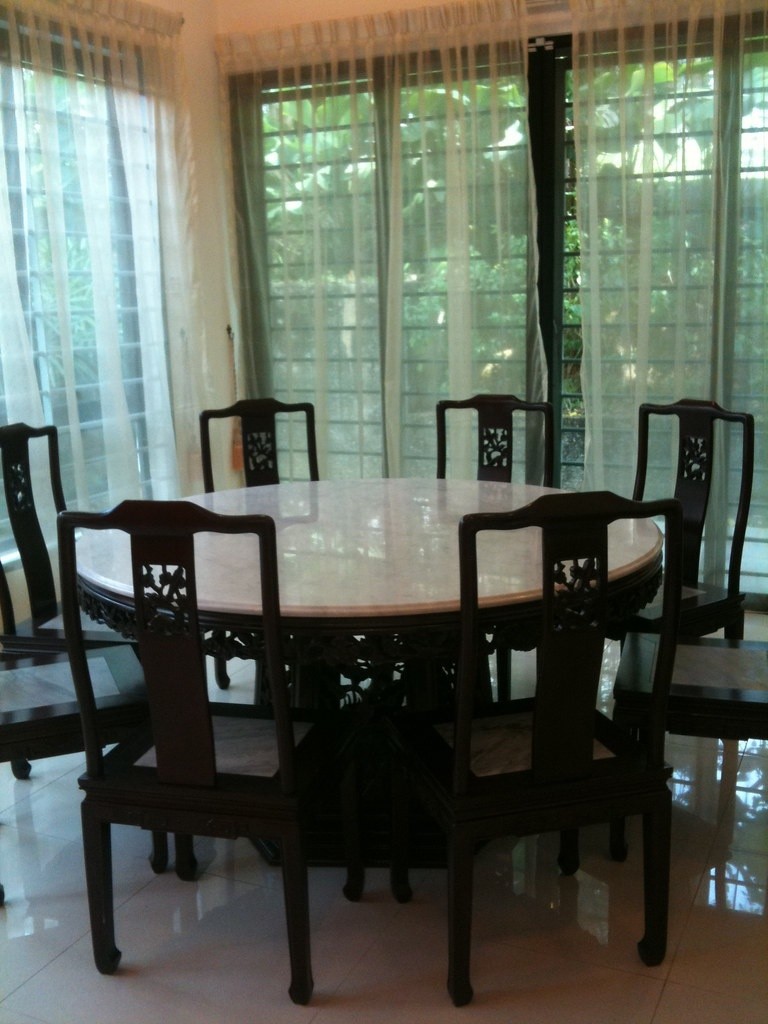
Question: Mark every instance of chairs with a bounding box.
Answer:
[0,393,768,1009]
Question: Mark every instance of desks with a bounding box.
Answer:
[70,477,665,913]
